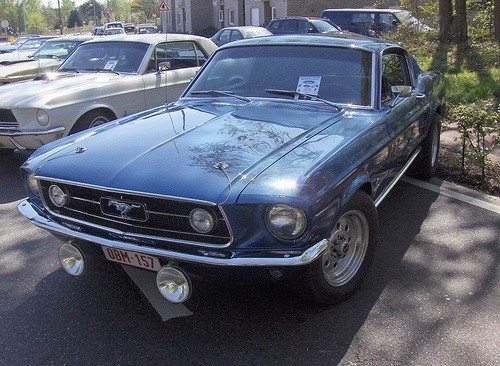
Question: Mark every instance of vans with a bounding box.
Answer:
[321,9,434,37]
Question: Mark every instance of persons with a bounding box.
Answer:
[105,14,112,22]
[262,24,266,28]
[8,26,14,36]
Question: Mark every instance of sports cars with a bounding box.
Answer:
[0,20,253,153]
[17,32,448,309]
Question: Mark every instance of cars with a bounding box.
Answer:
[206,24,272,49]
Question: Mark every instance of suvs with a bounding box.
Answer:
[262,14,344,36]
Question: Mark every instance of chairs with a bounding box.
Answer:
[258,52,382,110]
